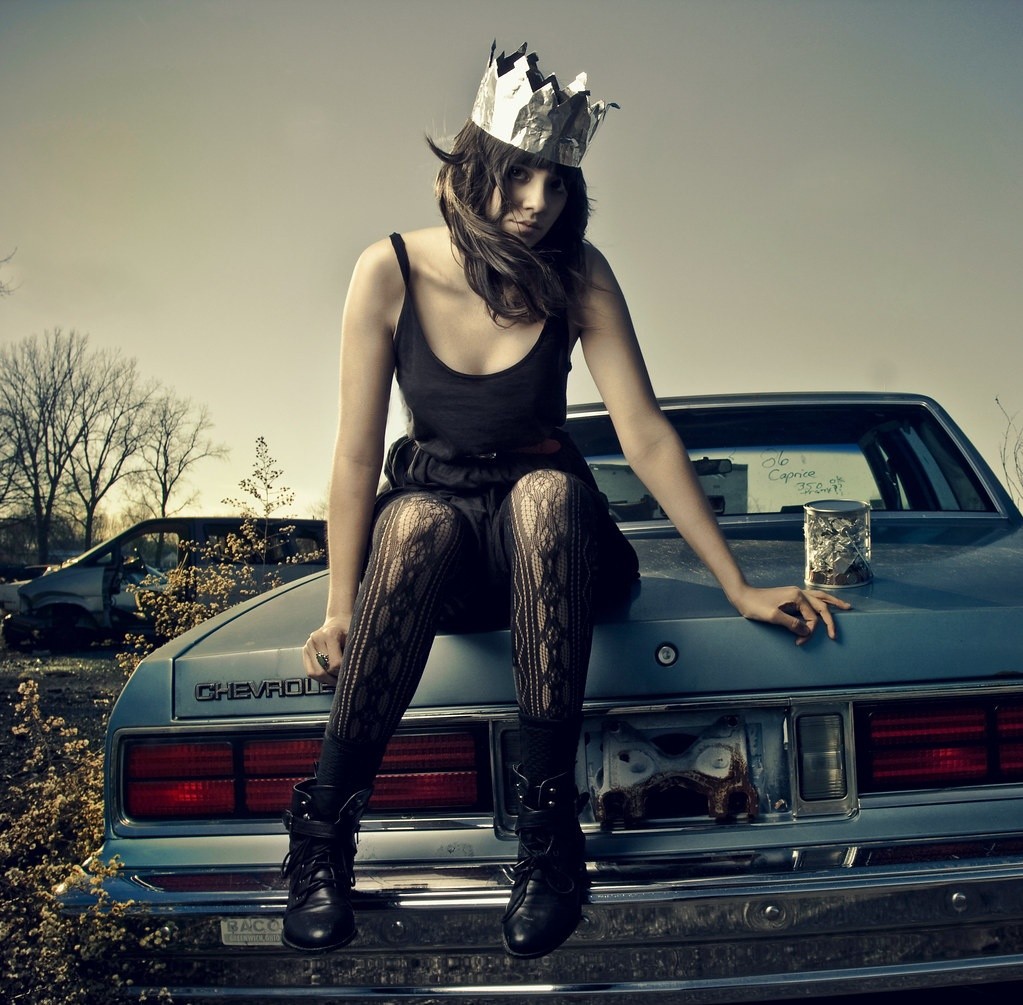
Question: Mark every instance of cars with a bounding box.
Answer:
[46,392,1023,997]
[0,559,61,613]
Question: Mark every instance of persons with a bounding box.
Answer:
[282,35,851,959]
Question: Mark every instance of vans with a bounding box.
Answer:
[3,517,330,657]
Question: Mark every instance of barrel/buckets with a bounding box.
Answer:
[801,499,876,589]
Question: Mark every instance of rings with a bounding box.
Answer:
[316,652,330,671]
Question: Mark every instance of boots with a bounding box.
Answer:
[500,763,590,960]
[281,778,374,954]
